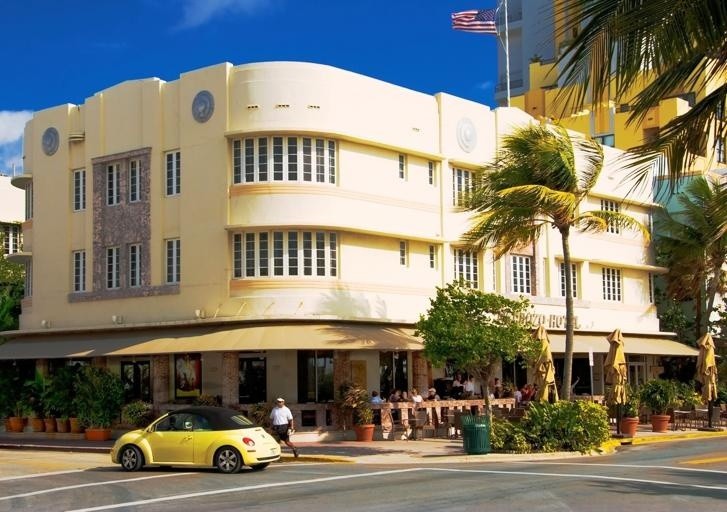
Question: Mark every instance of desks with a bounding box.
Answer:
[694,409,709,429]
[673,411,691,431]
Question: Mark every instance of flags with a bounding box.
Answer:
[450,7,497,34]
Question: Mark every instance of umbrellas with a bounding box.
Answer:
[603,329,626,434]
[530,324,560,406]
[694,332,717,427]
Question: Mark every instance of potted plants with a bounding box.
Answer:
[604,383,639,435]
[636,378,683,433]
[0,366,126,441]
[335,382,376,442]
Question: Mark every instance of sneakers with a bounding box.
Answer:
[293,446,299,458]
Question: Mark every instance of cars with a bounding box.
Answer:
[110,407,281,475]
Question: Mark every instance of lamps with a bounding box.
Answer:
[41,320,50,328]
[195,310,205,318]
[112,315,123,324]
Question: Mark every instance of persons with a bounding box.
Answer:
[369,374,536,425]
[268,396,301,457]
[167,415,178,431]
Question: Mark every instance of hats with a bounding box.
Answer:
[277,397,284,402]
[428,387,436,392]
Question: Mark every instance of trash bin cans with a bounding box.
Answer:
[461,415,490,455]
